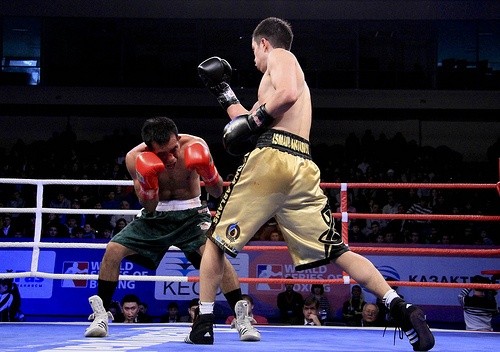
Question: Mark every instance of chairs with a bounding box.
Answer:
[59,201,142,239]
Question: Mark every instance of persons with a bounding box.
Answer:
[0,275,500,332]
[85,117,261,342]
[0,122,500,244]
[185,17,434,352]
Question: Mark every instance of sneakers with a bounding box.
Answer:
[233,300,262,341]
[85,294,114,338]
[383,298,435,351]
[185,305,214,345]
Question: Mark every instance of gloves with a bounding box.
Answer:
[134,151,168,200]
[183,142,219,186]
[223,104,276,155]
[198,57,240,109]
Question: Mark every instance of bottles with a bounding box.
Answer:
[321,310,327,319]
